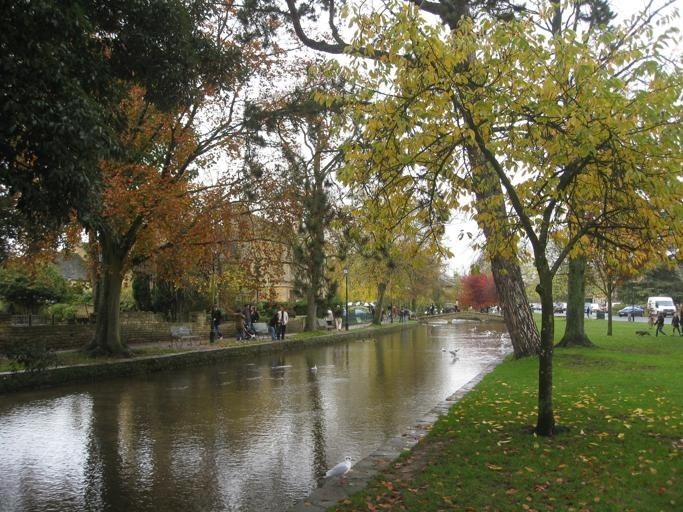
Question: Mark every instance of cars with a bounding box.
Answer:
[529,302,605,314]
[616,305,644,317]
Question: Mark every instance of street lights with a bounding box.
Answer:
[209,243,218,343]
[342,264,349,331]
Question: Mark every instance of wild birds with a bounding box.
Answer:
[449,347,460,357]
[322,455,357,484]
[441,346,445,352]
[310,363,319,370]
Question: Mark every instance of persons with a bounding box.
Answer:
[647,310,683,337]
[452,298,460,313]
[213,304,223,340]
[233,308,255,342]
[250,306,260,323]
[382,303,434,324]
[267,312,279,340]
[277,305,289,340]
[323,306,334,331]
[242,304,257,339]
[332,304,343,331]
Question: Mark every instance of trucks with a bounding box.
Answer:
[646,296,677,317]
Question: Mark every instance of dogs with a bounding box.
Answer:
[636,330,650,336]
